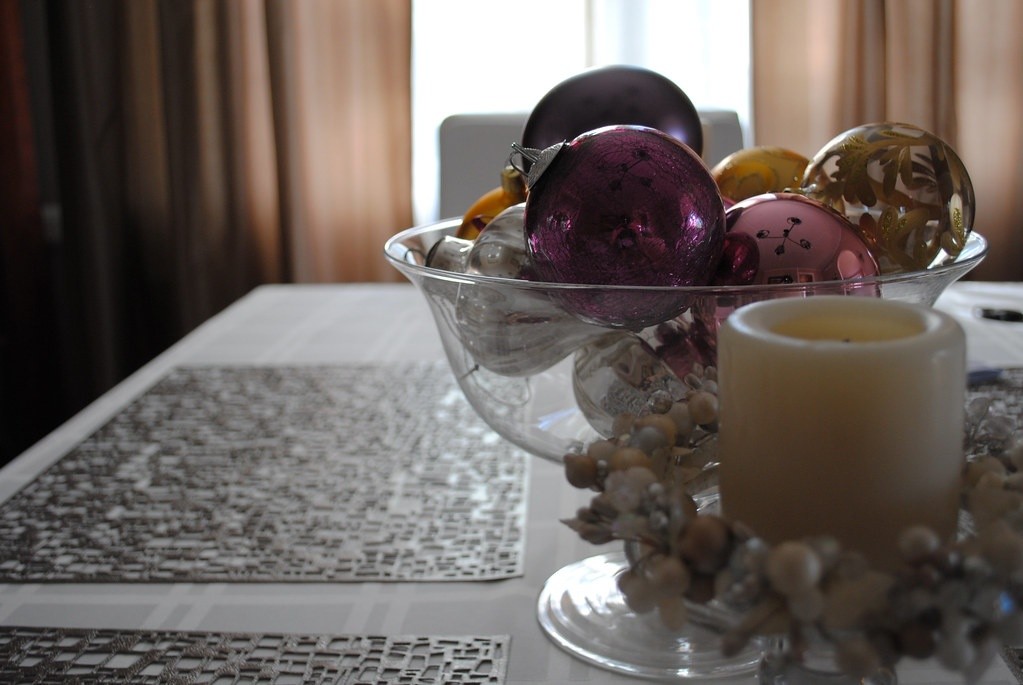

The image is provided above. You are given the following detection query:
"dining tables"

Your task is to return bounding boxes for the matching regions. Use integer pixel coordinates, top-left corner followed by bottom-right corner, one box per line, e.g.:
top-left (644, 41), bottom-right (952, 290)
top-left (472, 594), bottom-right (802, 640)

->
top-left (0, 286), bottom-right (1023, 685)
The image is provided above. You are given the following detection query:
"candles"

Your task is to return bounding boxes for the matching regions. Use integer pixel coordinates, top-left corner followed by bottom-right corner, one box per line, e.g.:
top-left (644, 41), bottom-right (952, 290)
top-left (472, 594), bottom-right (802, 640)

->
top-left (714, 293), bottom-right (968, 561)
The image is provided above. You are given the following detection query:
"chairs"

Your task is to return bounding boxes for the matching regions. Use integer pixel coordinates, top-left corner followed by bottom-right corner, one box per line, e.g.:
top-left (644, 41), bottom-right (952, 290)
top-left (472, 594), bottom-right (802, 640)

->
top-left (436, 111), bottom-right (744, 226)
